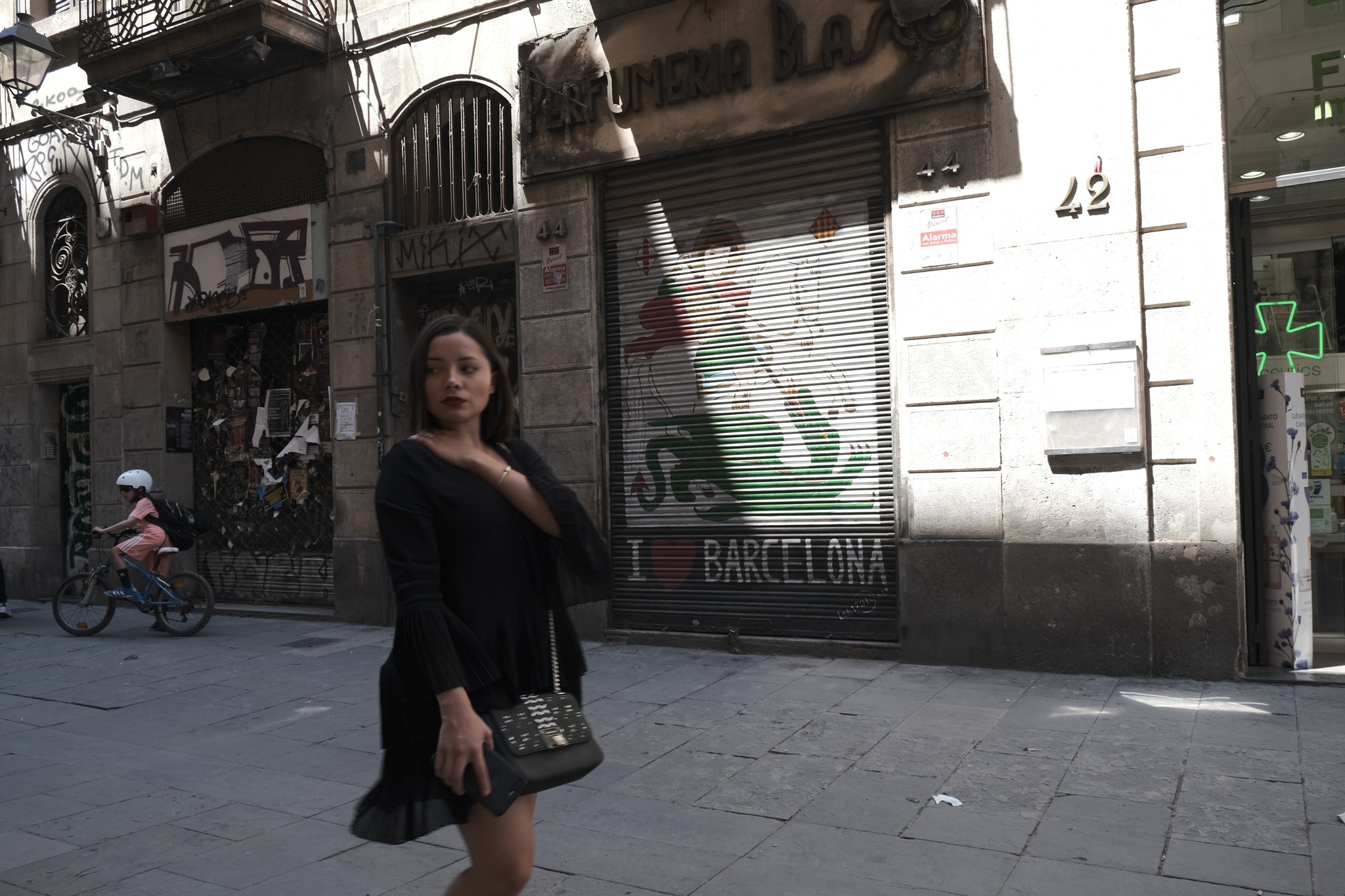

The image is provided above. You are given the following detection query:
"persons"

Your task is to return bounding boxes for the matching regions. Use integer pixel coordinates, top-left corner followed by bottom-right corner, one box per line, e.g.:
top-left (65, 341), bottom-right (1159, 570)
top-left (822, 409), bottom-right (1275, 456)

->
top-left (91, 469), bottom-right (172, 631)
top-left (349, 314), bottom-right (612, 896)
top-left (0, 562), bottom-right (12, 618)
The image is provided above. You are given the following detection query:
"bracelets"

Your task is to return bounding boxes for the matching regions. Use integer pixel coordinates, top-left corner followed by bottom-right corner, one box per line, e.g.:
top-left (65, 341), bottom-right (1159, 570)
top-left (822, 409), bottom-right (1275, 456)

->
top-left (495, 465), bottom-right (511, 489)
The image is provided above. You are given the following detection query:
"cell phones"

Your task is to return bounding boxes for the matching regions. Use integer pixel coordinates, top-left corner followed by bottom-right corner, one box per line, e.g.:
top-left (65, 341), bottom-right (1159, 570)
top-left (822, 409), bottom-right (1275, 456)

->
top-left (430, 743), bottom-right (528, 817)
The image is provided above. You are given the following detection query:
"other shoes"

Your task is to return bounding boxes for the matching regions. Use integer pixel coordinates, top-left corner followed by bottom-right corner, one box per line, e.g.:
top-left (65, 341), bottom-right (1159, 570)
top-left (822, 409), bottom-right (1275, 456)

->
top-left (0, 604), bottom-right (13, 617)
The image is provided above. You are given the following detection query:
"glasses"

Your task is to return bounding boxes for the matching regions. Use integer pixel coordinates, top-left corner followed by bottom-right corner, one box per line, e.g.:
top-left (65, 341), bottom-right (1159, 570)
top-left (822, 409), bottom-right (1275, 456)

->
top-left (119, 485), bottom-right (133, 493)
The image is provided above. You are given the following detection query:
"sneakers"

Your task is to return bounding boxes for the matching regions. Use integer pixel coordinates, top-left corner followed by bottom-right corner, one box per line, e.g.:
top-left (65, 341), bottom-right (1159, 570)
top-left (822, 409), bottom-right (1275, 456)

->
top-left (104, 586), bottom-right (133, 598)
top-left (147, 618), bottom-right (169, 630)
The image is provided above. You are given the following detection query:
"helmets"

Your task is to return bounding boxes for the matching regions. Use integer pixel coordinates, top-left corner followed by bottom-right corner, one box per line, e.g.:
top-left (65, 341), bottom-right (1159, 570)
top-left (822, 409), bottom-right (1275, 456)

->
top-left (116, 469), bottom-right (153, 493)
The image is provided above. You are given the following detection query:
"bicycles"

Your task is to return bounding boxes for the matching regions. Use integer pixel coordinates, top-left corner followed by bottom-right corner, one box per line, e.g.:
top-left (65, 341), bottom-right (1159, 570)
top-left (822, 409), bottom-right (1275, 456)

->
top-left (52, 526), bottom-right (215, 636)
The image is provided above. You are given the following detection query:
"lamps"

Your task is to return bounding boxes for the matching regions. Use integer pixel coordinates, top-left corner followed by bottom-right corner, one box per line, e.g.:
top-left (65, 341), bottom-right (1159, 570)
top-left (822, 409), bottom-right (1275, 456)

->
top-left (0, 13), bottom-right (105, 158)
top-left (239, 31), bottom-right (271, 67)
top-left (150, 58), bottom-right (181, 82)
top-left (83, 82), bottom-right (118, 108)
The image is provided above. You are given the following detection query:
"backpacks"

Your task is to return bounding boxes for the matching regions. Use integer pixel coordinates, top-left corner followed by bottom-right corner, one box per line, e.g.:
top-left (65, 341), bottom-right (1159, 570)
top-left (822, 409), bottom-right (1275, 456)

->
top-left (138, 494), bottom-right (196, 551)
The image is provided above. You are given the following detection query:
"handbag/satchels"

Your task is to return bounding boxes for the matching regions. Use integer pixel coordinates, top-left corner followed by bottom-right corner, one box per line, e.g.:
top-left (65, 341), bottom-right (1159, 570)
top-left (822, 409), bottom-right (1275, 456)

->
top-left (483, 691), bottom-right (603, 796)
